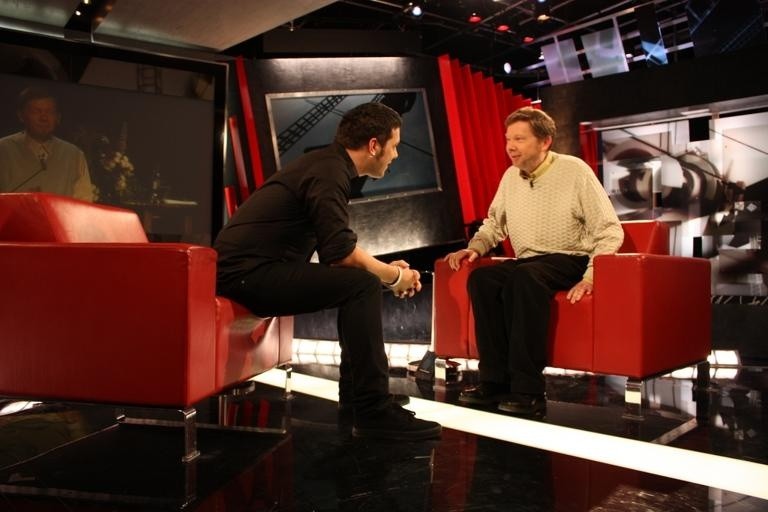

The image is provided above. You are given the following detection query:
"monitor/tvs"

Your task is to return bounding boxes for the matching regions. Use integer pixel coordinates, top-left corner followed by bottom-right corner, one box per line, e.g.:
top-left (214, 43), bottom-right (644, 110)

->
top-left (0, 23), bottom-right (230, 247)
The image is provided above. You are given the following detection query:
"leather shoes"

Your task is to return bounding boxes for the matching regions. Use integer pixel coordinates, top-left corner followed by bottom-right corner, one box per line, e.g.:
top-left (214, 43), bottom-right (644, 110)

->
top-left (338, 392), bottom-right (442, 440)
top-left (458, 384), bottom-right (548, 415)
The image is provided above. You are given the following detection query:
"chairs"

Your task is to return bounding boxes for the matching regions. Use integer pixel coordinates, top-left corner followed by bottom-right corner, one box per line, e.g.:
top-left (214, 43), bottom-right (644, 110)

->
top-left (433, 222), bottom-right (712, 424)
top-left (0, 192), bottom-right (295, 465)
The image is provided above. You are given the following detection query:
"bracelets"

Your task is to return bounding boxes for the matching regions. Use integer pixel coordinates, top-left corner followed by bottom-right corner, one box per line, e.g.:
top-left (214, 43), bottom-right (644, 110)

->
top-left (388, 265), bottom-right (404, 289)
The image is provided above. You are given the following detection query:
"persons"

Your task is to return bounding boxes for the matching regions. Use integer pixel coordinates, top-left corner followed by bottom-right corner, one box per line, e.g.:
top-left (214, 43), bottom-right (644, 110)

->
top-left (0, 86), bottom-right (93, 207)
top-left (214, 102), bottom-right (442, 441)
top-left (444, 106), bottom-right (624, 416)
